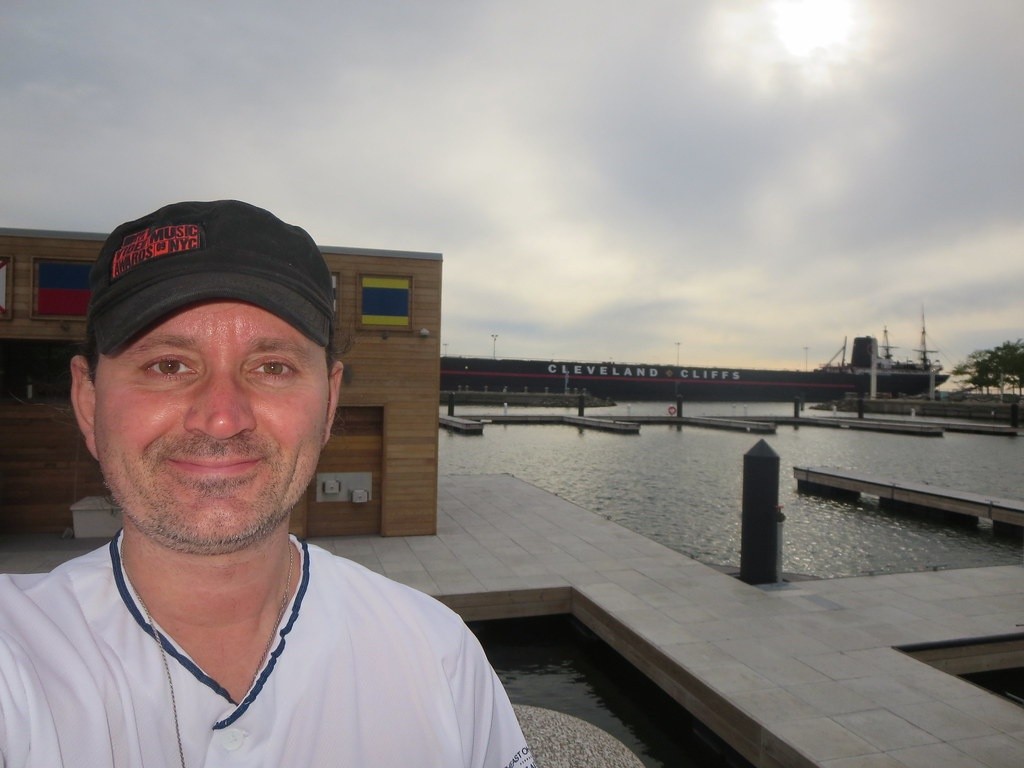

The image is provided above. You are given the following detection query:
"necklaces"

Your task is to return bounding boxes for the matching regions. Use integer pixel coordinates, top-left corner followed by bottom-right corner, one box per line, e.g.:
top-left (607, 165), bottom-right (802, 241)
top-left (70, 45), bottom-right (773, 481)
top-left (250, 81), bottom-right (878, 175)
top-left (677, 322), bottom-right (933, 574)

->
top-left (117, 536), bottom-right (292, 768)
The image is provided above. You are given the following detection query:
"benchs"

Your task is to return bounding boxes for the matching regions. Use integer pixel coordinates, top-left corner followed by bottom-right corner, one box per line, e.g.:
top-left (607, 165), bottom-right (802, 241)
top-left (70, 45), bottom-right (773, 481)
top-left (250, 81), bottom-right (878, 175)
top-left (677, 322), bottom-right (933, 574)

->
top-left (70, 496), bottom-right (121, 538)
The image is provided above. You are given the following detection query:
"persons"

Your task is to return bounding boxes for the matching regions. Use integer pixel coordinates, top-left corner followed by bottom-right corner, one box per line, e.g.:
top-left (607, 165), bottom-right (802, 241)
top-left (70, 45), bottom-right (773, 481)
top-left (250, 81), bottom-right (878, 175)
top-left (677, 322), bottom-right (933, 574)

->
top-left (2, 200), bottom-right (540, 768)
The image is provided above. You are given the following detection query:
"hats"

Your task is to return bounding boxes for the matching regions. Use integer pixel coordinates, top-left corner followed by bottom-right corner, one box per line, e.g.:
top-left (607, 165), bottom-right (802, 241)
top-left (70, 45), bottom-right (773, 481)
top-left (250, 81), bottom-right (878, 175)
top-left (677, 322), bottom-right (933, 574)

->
top-left (84, 200), bottom-right (334, 363)
